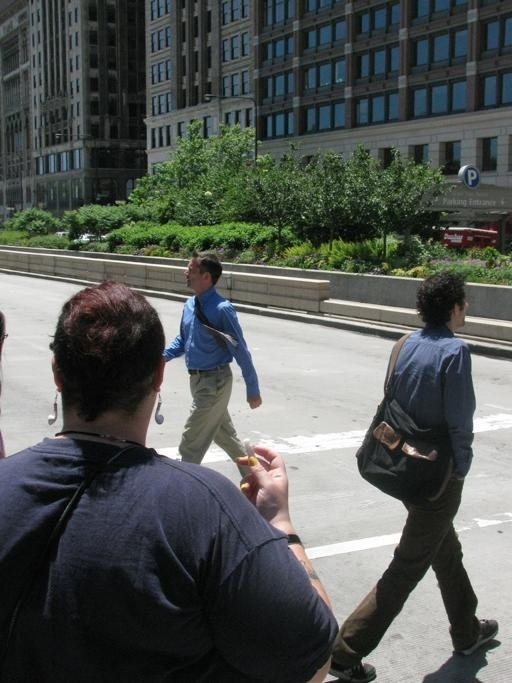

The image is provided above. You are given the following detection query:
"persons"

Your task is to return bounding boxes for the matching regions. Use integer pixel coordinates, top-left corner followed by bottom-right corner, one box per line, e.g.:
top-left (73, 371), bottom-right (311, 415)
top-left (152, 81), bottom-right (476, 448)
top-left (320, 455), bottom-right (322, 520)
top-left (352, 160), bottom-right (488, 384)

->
top-left (0, 310), bottom-right (9, 459)
top-left (0, 278), bottom-right (341, 681)
top-left (159, 251), bottom-right (262, 478)
top-left (326, 268), bottom-right (501, 683)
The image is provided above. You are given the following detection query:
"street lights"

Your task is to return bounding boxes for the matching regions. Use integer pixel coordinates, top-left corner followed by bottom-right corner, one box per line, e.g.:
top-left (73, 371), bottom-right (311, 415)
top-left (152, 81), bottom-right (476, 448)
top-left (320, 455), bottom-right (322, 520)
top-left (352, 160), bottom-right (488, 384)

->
top-left (56, 132), bottom-right (101, 201)
top-left (204, 92), bottom-right (258, 164)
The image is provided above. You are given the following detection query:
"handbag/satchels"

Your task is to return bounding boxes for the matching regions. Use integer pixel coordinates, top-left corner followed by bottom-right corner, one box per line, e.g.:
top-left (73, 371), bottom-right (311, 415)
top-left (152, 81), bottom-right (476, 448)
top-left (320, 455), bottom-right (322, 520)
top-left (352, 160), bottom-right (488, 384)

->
top-left (355, 393), bottom-right (450, 501)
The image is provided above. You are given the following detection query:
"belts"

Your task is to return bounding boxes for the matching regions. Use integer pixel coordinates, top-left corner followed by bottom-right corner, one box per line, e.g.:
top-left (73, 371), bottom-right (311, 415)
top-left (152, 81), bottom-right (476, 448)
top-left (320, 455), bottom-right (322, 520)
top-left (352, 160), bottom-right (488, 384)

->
top-left (187, 368), bottom-right (205, 374)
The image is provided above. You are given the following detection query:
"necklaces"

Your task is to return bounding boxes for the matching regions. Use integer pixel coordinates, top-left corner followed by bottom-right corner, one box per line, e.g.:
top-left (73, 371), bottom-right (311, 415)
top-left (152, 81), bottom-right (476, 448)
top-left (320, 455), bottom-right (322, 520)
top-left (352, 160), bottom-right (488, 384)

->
top-left (54, 430), bottom-right (148, 448)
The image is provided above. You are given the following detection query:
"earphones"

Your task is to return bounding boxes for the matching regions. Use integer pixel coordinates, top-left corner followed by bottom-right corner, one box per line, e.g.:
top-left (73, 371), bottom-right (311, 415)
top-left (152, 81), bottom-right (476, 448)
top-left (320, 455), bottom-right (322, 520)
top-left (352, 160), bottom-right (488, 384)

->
top-left (47, 403), bottom-right (59, 425)
top-left (155, 400), bottom-right (164, 427)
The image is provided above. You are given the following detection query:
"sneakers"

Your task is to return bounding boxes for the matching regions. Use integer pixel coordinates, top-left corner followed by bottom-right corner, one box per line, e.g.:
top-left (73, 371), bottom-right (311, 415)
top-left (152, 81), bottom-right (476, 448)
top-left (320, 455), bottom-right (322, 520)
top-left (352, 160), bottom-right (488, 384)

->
top-left (455, 620), bottom-right (498, 656)
top-left (329, 662), bottom-right (376, 682)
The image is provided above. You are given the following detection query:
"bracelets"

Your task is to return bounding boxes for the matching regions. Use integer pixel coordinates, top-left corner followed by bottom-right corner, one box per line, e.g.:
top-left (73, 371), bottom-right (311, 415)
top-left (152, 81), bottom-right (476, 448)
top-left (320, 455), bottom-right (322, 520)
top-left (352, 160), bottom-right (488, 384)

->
top-left (283, 533), bottom-right (303, 544)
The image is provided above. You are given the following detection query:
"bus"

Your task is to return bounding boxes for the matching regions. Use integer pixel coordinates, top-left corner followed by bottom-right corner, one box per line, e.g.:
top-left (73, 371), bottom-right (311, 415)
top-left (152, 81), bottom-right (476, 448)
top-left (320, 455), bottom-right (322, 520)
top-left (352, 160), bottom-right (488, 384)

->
top-left (429, 227), bottom-right (500, 252)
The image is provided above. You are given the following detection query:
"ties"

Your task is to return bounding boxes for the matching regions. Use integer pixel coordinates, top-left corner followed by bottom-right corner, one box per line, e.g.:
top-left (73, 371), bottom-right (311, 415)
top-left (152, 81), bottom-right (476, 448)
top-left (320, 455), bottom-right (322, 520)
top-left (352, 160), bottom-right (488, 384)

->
top-left (194, 296), bottom-right (238, 351)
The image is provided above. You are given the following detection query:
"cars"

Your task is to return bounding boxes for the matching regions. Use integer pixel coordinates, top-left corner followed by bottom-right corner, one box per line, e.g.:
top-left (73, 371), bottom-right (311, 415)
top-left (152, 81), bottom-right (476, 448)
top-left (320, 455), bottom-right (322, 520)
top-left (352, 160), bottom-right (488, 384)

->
top-left (55, 231), bottom-right (107, 245)
